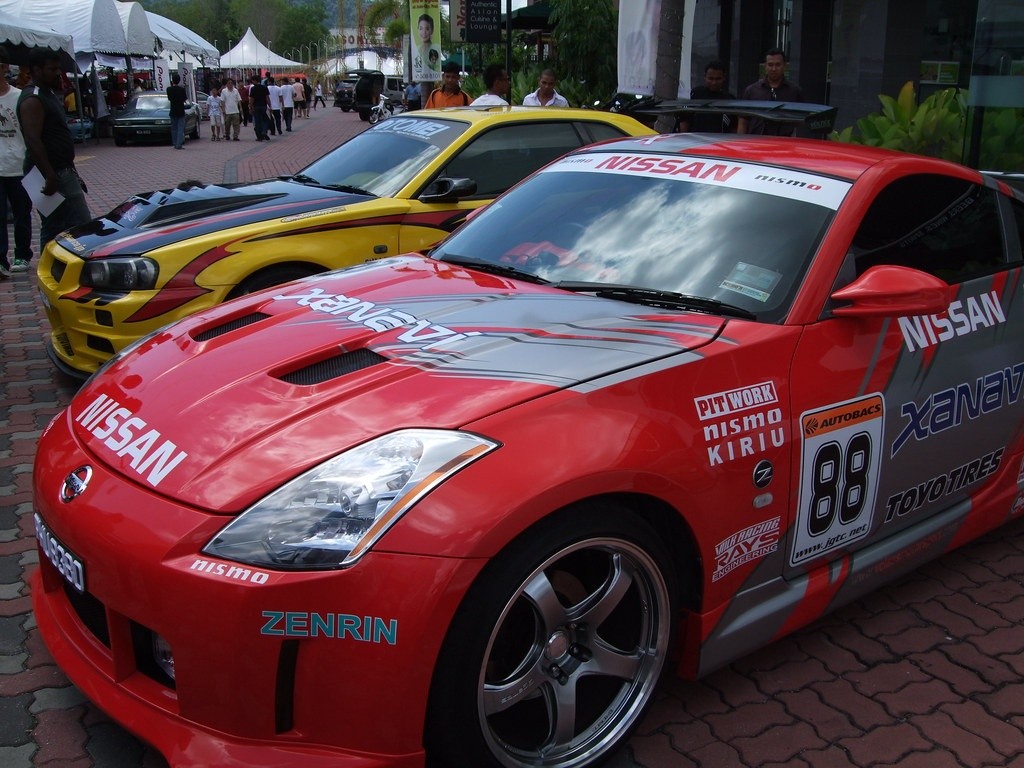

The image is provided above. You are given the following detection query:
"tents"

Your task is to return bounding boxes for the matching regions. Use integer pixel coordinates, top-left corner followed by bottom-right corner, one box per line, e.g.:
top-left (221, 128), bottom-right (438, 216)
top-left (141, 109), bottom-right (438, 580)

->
top-left (0, 0), bottom-right (221, 146)
top-left (195, 27), bottom-right (308, 69)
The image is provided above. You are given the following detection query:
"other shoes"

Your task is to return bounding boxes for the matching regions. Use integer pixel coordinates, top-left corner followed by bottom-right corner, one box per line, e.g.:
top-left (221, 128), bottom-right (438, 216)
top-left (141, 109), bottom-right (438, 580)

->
top-left (226, 136), bottom-right (230, 140)
top-left (271, 132), bottom-right (275, 135)
top-left (286, 128), bottom-right (291, 131)
top-left (256, 138), bottom-right (261, 141)
top-left (278, 129), bottom-right (282, 134)
top-left (211, 135), bottom-right (216, 141)
top-left (233, 138), bottom-right (239, 141)
top-left (216, 137), bottom-right (220, 141)
top-left (263, 133), bottom-right (270, 140)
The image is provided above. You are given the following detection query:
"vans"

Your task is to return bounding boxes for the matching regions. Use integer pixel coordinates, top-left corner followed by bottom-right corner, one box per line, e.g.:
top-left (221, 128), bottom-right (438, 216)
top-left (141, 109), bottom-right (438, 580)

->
top-left (345, 69), bottom-right (407, 120)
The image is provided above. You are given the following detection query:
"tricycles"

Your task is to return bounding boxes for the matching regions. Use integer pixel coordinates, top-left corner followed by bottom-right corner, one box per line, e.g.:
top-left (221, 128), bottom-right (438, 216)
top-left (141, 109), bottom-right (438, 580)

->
top-left (583, 92), bottom-right (839, 142)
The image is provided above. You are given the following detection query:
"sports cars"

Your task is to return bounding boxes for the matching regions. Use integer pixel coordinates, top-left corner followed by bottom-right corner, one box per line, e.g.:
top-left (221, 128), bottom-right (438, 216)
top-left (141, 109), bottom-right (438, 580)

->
top-left (37, 101), bottom-right (664, 376)
top-left (28, 131), bottom-right (1024, 768)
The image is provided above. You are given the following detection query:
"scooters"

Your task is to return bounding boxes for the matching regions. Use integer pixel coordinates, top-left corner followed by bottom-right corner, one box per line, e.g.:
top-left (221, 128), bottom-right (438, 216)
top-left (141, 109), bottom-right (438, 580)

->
top-left (368, 92), bottom-right (395, 124)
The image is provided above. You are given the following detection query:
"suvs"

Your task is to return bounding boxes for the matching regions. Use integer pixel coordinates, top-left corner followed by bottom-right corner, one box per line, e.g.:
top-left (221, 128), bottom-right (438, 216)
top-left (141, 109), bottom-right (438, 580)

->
top-left (332, 77), bottom-right (357, 113)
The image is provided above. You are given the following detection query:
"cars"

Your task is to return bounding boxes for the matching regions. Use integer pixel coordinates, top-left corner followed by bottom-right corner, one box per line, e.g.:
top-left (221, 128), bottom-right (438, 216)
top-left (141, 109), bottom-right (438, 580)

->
top-left (112, 90), bottom-right (224, 146)
top-left (65, 114), bottom-right (95, 143)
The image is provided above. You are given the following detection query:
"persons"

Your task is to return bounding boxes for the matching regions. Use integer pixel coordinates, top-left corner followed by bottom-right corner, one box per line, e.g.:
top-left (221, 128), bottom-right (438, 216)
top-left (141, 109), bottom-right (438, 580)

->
top-left (167, 74), bottom-right (188, 149)
top-left (737, 48), bottom-right (803, 136)
top-left (424, 61), bottom-right (474, 109)
top-left (0, 46), bottom-right (91, 278)
top-left (680, 61), bottom-right (738, 133)
top-left (523, 69), bottom-right (569, 108)
top-left (470, 64), bottom-right (510, 106)
top-left (206, 72), bottom-right (326, 141)
top-left (417, 14), bottom-right (441, 72)
top-left (405, 79), bottom-right (421, 111)
top-left (131, 78), bottom-right (142, 93)
top-left (426, 49), bottom-right (440, 72)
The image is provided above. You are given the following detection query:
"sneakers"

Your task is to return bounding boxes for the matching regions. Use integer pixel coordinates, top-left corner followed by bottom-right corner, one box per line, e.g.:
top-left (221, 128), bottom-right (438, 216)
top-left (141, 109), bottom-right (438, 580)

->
top-left (10, 259), bottom-right (31, 270)
top-left (0, 265), bottom-right (10, 276)
